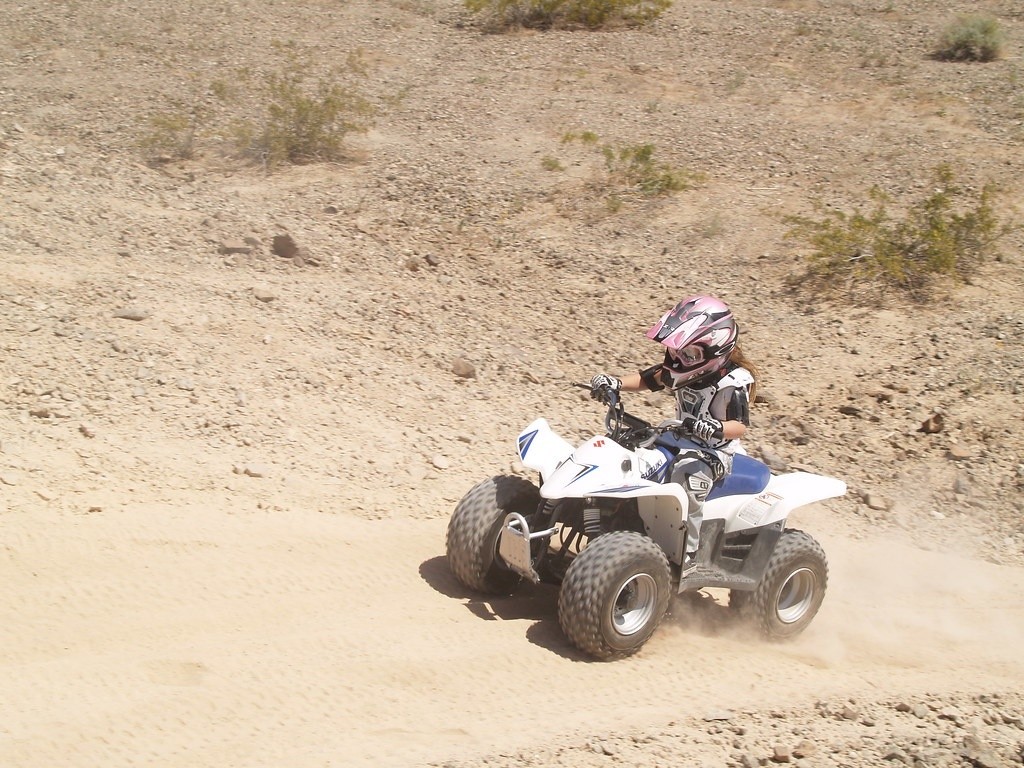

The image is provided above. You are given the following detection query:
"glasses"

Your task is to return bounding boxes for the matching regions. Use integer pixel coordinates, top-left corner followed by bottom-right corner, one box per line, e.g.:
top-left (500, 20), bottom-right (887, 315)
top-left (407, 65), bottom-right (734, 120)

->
top-left (667, 345), bottom-right (705, 367)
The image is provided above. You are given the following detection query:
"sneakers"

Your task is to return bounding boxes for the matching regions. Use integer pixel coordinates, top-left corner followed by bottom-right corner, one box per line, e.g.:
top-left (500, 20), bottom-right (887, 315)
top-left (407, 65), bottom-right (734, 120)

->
top-left (682, 553), bottom-right (697, 578)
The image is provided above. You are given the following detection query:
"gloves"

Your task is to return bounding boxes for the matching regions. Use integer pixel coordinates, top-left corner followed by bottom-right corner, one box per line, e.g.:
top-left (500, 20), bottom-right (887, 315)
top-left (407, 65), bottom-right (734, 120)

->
top-left (589, 373), bottom-right (623, 402)
top-left (687, 418), bottom-right (723, 446)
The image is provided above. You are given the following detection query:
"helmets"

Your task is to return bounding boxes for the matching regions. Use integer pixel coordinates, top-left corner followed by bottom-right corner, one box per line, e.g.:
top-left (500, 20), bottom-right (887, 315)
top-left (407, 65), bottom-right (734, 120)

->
top-left (646, 296), bottom-right (738, 391)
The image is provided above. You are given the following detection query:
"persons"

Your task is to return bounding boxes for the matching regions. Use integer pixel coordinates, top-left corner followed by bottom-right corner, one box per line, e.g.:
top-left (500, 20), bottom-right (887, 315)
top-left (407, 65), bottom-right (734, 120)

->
top-left (590, 294), bottom-right (761, 578)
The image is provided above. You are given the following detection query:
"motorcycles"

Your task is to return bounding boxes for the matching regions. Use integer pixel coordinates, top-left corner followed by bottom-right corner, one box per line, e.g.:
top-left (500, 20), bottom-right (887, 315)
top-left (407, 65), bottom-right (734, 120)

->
top-left (445, 382), bottom-right (848, 664)
